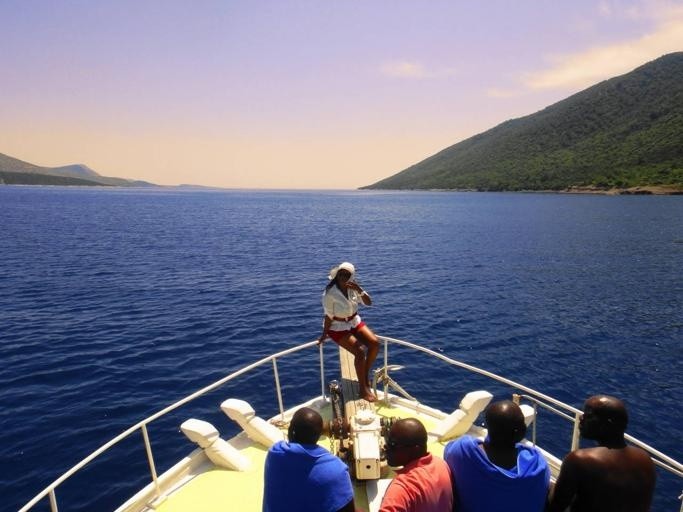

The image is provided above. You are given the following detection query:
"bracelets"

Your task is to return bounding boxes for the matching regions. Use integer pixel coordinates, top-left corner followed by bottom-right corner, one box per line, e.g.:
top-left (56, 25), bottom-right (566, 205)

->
top-left (358, 290), bottom-right (366, 297)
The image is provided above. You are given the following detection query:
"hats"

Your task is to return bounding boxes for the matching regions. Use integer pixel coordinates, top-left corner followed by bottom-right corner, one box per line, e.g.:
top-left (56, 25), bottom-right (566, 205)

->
top-left (330, 261), bottom-right (356, 282)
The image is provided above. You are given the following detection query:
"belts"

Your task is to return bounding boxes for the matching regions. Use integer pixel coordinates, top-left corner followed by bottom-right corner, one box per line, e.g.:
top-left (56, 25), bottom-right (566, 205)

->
top-left (333, 311), bottom-right (357, 322)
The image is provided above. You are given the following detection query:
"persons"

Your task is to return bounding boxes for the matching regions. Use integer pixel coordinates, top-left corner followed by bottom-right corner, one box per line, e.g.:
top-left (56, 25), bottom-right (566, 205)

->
top-left (317, 261), bottom-right (380, 402)
top-left (379, 418), bottom-right (454, 512)
top-left (444, 400), bottom-right (550, 512)
top-left (549, 395), bottom-right (657, 512)
top-left (262, 407), bottom-right (356, 512)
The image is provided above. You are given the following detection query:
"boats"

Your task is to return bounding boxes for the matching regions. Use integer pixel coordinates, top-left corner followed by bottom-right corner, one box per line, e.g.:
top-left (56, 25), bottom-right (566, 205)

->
top-left (16, 331), bottom-right (682, 510)
